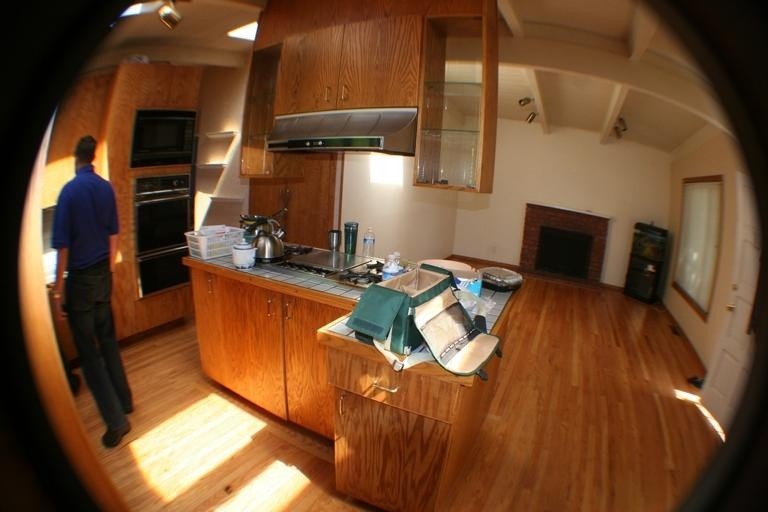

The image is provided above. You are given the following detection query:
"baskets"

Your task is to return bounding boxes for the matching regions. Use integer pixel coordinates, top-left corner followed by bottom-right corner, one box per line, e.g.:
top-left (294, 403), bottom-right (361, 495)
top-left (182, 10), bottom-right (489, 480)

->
top-left (184, 226), bottom-right (247, 260)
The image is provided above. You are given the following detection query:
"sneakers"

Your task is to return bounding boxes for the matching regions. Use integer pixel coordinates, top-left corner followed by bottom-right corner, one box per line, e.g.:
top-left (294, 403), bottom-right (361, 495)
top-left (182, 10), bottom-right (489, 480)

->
top-left (102, 405), bottom-right (133, 447)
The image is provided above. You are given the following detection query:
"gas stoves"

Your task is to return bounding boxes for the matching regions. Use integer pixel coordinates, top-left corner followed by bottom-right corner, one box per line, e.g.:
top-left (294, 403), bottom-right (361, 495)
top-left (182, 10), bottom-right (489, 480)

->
top-left (264, 244), bottom-right (418, 289)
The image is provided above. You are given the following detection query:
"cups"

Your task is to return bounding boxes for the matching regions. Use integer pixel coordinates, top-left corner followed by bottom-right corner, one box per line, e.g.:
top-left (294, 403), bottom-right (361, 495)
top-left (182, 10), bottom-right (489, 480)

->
top-left (344, 222), bottom-right (358, 254)
top-left (328, 229), bottom-right (341, 252)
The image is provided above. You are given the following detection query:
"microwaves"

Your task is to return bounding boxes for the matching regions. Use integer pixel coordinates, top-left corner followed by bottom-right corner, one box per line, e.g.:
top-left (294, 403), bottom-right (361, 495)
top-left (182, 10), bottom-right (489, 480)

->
top-left (132, 110), bottom-right (197, 166)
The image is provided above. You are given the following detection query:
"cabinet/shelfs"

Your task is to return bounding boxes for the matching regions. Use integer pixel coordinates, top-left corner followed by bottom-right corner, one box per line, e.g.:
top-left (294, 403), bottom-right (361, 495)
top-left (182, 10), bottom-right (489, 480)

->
top-left (230, 281), bottom-right (348, 442)
top-left (413, 6), bottom-right (498, 193)
top-left (238, 43), bottom-right (306, 180)
top-left (188, 268), bottom-right (227, 386)
top-left (276, 16), bottom-right (419, 118)
top-left (315, 274), bottom-right (530, 512)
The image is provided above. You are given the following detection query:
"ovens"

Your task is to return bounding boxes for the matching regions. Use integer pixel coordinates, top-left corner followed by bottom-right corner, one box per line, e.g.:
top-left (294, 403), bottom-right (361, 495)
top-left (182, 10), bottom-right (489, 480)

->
top-left (132, 173), bottom-right (193, 298)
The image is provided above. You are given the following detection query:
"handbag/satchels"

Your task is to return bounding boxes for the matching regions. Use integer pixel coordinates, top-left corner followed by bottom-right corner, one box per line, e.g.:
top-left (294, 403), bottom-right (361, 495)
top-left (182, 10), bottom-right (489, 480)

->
top-left (346, 264), bottom-right (500, 375)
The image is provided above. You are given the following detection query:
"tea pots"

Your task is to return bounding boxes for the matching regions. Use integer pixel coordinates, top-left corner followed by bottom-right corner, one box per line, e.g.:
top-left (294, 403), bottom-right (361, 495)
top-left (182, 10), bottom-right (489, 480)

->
top-left (251, 228), bottom-right (287, 264)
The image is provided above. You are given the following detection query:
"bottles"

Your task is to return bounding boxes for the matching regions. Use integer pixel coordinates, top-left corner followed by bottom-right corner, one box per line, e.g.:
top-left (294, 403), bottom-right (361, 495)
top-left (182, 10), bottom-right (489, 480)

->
top-left (362, 227), bottom-right (376, 258)
top-left (230, 237), bottom-right (258, 269)
top-left (382, 252), bottom-right (401, 275)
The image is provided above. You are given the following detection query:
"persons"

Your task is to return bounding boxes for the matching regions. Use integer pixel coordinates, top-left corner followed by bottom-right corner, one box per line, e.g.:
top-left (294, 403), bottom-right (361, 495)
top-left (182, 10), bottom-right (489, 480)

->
top-left (51, 136), bottom-right (134, 448)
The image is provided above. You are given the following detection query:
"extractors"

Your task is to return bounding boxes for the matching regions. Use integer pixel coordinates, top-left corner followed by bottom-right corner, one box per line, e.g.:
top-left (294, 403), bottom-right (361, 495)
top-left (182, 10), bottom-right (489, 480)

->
top-left (264, 103), bottom-right (419, 159)
